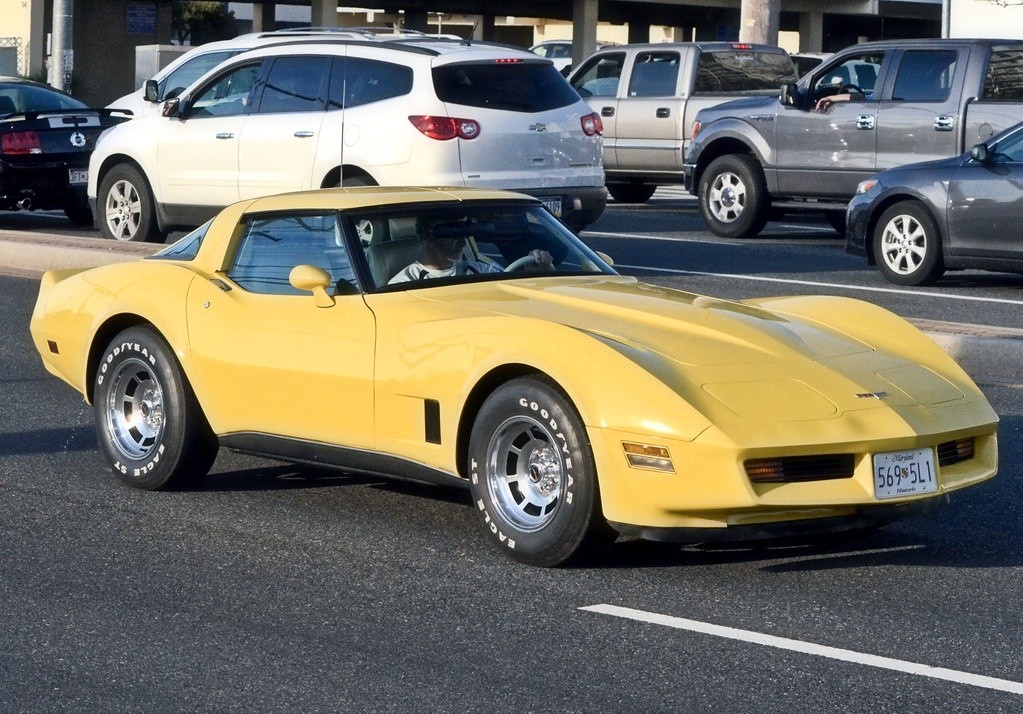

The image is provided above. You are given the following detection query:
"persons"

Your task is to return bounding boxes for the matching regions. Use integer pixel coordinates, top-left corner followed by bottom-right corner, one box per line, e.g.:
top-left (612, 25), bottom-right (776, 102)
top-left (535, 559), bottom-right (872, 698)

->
top-left (814, 94), bottom-right (872, 113)
top-left (386, 216), bottom-right (553, 286)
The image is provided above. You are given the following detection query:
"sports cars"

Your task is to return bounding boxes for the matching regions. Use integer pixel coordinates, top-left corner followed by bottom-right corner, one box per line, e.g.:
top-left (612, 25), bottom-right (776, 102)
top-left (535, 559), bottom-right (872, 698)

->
top-left (30, 42), bottom-right (1000, 568)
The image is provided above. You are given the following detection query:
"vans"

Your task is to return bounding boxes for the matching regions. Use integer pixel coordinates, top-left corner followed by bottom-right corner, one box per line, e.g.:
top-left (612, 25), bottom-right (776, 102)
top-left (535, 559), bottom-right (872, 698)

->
top-left (87, 27), bottom-right (607, 269)
top-left (567, 42), bottom-right (802, 203)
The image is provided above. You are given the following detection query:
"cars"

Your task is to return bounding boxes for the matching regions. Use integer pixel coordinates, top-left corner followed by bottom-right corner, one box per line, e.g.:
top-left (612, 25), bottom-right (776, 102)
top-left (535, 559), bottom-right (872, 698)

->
top-left (843, 121), bottom-right (1023, 286)
top-left (0, 76), bottom-right (135, 230)
top-left (790, 52), bottom-right (835, 78)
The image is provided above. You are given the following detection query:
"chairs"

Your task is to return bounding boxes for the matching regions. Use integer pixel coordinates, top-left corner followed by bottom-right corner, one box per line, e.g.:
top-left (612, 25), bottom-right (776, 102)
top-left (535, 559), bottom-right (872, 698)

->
top-left (368, 239), bottom-right (419, 288)
top-left (0, 95), bottom-right (17, 115)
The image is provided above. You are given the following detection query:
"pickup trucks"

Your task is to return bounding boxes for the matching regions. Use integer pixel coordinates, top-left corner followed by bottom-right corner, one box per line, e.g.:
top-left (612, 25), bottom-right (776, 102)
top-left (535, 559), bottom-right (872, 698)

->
top-left (684, 38), bottom-right (1023, 238)
